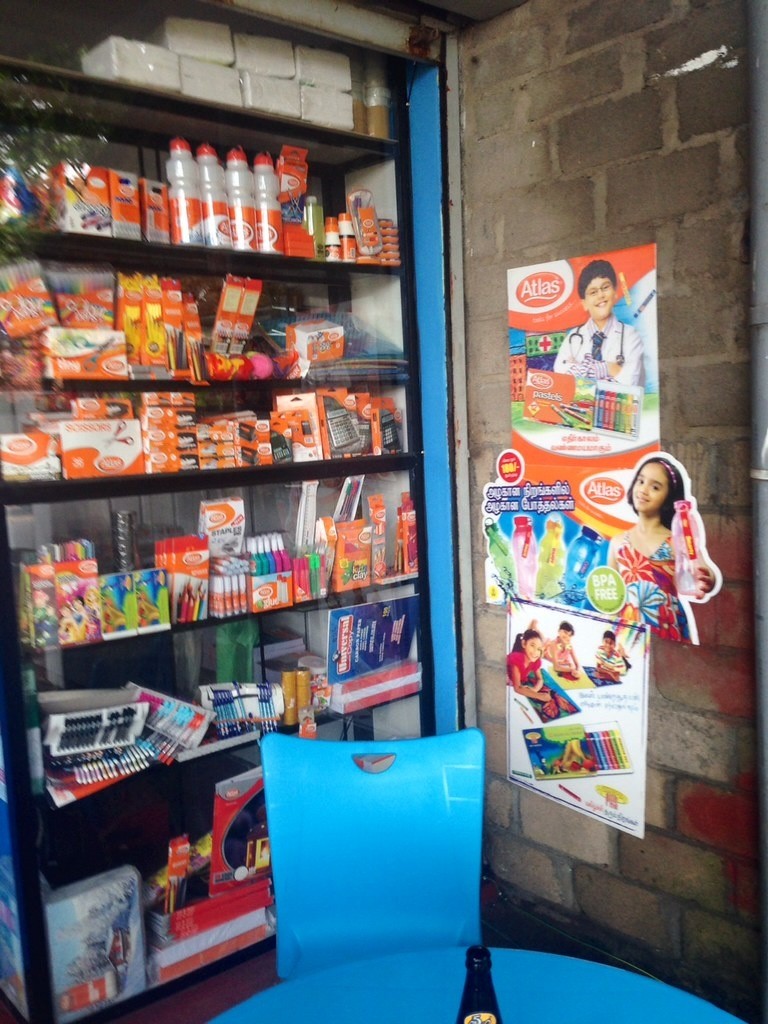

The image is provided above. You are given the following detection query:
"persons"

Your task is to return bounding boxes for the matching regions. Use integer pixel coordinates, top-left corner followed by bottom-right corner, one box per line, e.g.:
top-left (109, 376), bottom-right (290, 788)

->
top-left (506, 629), bottom-right (552, 702)
top-left (528, 619), bottom-right (582, 679)
top-left (607, 456), bottom-right (716, 646)
top-left (595, 630), bottom-right (627, 682)
top-left (553, 260), bottom-right (645, 387)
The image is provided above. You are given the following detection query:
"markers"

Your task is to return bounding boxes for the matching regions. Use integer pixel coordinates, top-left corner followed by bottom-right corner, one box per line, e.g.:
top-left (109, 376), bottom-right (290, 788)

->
top-left (557, 783), bottom-right (582, 803)
top-left (514, 697), bottom-right (534, 724)
top-left (293, 553), bottom-right (326, 603)
top-left (584, 729), bottom-right (631, 770)
top-left (207, 556), bottom-right (249, 618)
top-left (512, 770), bottom-right (532, 779)
top-left (618, 271), bottom-right (656, 318)
top-left (550, 389), bottom-right (641, 438)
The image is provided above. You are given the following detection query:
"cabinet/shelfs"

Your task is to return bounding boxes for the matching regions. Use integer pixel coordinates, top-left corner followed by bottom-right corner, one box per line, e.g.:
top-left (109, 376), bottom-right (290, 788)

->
top-left (0, 43), bottom-right (439, 1024)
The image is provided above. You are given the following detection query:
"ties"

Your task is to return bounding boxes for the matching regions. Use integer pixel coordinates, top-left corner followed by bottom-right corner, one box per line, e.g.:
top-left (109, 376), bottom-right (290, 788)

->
top-left (591, 332), bottom-right (605, 362)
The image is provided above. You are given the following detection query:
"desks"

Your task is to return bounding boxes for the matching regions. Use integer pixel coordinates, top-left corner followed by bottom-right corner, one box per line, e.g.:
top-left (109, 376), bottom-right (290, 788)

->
top-left (201, 945), bottom-right (749, 1023)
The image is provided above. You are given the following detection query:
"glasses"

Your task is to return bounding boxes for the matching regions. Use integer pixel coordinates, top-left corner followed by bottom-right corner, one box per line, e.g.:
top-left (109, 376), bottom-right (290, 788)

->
top-left (584, 282), bottom-right (613, 296)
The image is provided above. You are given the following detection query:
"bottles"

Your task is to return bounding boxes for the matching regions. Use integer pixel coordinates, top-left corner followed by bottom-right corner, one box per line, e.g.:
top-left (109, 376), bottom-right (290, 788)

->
top-left (484, 517), bottom-right (515, 582)
top-left (253, 150), bottom-right (283, 254)
top-left (455, 945), bottom-right (504, 1024)
top-left (346, 185), bottom-right (382, 255)
top-left (337, 212), bottom-right (357, 263)
top-left (195, 140), bottom-right (231, 249)
top-left (303, 194), bottom-right (327, 262)
top-left (563, 525), bottom-right (601, 601)
top-left (671, 499), bottom-right (708, 596)
top-left (536, 513), bottom-right (566, 598)
top-left (225, 144), bottom-right (256, 253)
top-left (166, 135), bottom-right (206, 247)
top-left (323, 217), bottom-right (342, 262)
top-left (511, 516), bottom-right (538, 597)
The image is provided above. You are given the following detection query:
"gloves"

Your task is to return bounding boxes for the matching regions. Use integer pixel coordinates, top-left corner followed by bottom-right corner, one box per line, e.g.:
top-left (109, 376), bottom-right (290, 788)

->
top-left (568, 354), bottom-right (610, 380)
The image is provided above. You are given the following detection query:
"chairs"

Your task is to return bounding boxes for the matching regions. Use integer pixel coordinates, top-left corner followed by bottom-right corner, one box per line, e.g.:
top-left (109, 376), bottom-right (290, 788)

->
top-left (258, 724), bottom-right (485, 982)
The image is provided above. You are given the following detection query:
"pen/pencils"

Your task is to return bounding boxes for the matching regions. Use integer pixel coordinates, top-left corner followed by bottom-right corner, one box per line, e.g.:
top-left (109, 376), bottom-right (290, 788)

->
top-left (336, 479), bottom-right (359, 522)
top-left (123, 302), bottom-right (212, 382)
top-left (177, 577), bottom-right (207, 622)
top-left (394, 492), bottom-right (418, 575)
top-left (44, 691), bottom-right (206, 785)
top-left (0, 257), bottom-right (115, 294)
top-left (211, 681), bottom-right (279, 748)
top-left (18, 538), bottom-right (94, 566)
top-left (214, 330), bottom-right (250, 355)
top-left (165, 872), bottom-right (188, 914)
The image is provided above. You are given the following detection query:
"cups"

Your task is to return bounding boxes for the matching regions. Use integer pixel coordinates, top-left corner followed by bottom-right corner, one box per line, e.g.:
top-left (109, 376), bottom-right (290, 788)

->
top-left (349, 82), bottom-right (367, 133)
top-left (366, 87), bottom-right (391, 140)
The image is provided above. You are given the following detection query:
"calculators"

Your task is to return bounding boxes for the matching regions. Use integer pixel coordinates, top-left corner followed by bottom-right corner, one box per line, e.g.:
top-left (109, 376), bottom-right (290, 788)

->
top-left (382, 413), bottom-right (399, 447)
top-left (301, 420), bottom-right (312, 435)
top-left (269, 432), bottom-right (291, 461)
top-left (327, 407), bottom-right (372, 454)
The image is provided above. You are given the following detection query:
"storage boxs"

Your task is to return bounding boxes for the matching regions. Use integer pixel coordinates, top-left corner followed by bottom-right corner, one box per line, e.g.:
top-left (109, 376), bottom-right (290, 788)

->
top-left (1, 145), bottom-right (405, 655)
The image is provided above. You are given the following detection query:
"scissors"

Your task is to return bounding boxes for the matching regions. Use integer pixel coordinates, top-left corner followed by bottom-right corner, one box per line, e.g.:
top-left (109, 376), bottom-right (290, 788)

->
top-left (99, 421), bottom-right (135, 451)
top-left (73, 336), bottom-right (125, 371)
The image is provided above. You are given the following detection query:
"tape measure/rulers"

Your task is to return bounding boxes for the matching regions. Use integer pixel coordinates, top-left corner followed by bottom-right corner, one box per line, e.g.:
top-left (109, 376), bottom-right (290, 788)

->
top-left (300, 483), bottom-right (318, 556)
